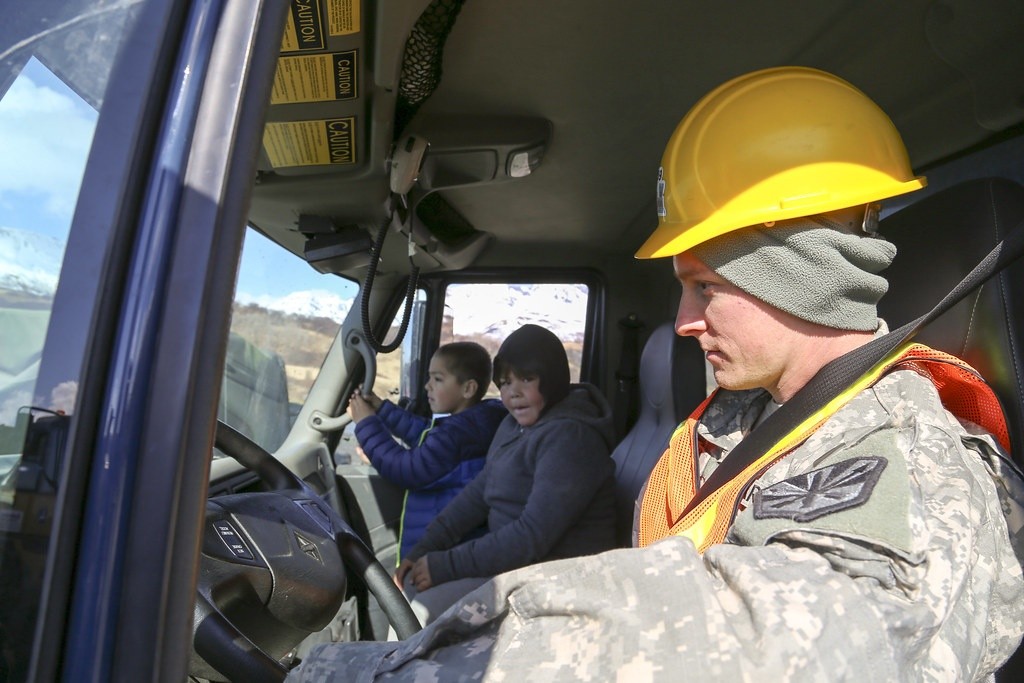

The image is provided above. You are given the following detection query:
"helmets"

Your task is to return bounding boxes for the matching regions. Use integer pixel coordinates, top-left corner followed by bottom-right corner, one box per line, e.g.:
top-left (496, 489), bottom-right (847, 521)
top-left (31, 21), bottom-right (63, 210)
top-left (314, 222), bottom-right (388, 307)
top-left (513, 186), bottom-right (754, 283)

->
top-left (633, 64), bottom-right (927, 259)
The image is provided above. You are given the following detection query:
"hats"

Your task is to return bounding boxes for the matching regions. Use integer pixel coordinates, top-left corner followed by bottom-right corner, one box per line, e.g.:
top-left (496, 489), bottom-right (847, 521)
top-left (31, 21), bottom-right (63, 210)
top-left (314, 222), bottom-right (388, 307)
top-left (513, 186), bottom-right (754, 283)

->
top-left (498, 325), bottom-right (570, 394)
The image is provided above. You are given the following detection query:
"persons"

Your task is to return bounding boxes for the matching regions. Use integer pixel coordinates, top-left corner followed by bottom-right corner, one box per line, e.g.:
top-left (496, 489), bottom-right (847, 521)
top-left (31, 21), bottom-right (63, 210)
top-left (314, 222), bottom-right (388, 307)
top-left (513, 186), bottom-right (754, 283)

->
top-left (383, 322), bottom-right (623, 648)
top-left (351, 339), bottom-right (510, 644)
top-left (283, 65), bottom-right (1024, 683)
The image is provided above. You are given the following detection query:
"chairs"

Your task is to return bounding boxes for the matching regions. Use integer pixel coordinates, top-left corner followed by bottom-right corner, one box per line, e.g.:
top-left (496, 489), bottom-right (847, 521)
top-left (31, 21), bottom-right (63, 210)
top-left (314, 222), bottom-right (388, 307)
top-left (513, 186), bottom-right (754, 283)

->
top-left (607, 324), bottom-right (706, 512)
top-left (876, 163), bottom-right (1024, 470)
